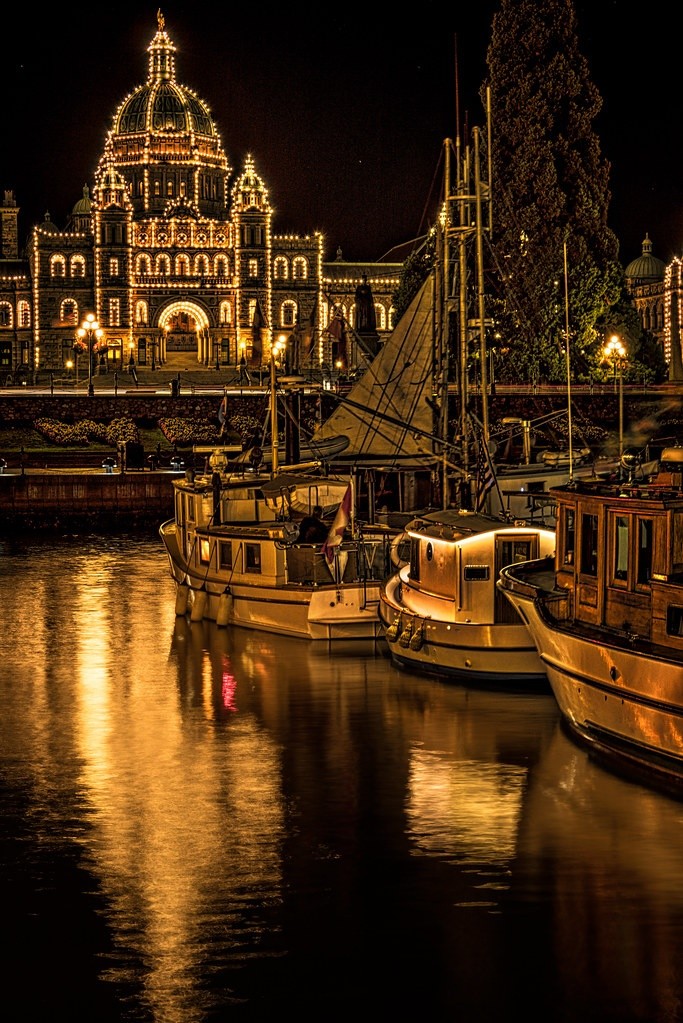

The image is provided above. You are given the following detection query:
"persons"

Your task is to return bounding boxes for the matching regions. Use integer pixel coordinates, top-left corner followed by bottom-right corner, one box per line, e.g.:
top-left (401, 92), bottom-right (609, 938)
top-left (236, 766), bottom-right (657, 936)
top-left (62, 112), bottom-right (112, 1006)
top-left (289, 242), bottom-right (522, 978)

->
top-left (291, 506), bottom-right (326, 548)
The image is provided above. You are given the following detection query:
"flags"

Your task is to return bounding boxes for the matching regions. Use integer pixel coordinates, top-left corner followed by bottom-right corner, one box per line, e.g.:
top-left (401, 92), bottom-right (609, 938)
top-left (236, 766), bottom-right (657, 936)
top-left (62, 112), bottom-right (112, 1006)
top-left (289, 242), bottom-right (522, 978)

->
top-left (321, 484), bottom-right (352, 563)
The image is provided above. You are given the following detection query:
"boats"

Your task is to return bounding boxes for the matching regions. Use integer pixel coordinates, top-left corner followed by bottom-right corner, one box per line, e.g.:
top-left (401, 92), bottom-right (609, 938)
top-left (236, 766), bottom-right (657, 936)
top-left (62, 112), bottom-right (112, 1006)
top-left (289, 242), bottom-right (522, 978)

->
top-left (158, 333), bottom-right (392, 641)
top-left (376, 501), bottom-right (551, 689)
top-left (234, 434), bottom-right (350, 465)
top-left (496, 440), bottom-right (683, 782)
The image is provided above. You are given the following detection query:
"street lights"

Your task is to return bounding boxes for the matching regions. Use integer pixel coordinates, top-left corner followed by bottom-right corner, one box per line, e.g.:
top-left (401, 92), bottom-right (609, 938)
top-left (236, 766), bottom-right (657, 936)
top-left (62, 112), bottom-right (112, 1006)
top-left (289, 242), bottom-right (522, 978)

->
top-left (272, 335), bottom-right (288, 387)
top-left (337, 363), bottom-right (342, 376)
top-left (77, 313), bottom-right (104, 396)
top-left (129, 343), bottom-right (135, 365)
top-left (601, 335), bottom-right (626, 392)
top-left (240, 342), bottom-right (246, 365)
top-left (67, 362), bottom-right (73, 374)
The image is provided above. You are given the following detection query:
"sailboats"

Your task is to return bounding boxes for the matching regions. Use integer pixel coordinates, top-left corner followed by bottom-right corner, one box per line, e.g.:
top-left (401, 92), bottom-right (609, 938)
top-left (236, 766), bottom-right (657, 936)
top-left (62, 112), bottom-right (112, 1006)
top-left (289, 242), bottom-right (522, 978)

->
top-left (306, 81), bottom-right (621, 523)
